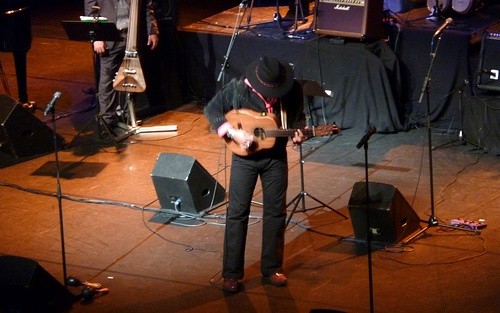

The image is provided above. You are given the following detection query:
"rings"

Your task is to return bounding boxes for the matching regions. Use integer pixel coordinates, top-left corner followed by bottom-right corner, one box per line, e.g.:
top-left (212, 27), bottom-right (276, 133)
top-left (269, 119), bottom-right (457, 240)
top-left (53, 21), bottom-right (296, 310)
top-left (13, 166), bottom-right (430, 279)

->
top-left (246, 144), bottom-right (250, 147)
top-left (246, 141), bottom-right (250, 143)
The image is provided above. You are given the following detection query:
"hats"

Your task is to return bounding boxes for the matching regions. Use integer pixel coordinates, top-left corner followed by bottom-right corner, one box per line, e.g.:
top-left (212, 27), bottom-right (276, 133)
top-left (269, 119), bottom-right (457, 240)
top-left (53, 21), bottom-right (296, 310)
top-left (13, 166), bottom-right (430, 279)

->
top-left (245, 54), bottom-right (294, 97)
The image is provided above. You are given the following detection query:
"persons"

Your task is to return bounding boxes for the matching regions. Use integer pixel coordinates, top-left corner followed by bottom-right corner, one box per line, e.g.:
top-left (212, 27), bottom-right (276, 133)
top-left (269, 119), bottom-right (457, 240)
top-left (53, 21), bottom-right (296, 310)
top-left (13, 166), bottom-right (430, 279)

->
top-left (202, 56), bottom-right (312, 292)
top-left (84, 0), bottom-right (159, 146)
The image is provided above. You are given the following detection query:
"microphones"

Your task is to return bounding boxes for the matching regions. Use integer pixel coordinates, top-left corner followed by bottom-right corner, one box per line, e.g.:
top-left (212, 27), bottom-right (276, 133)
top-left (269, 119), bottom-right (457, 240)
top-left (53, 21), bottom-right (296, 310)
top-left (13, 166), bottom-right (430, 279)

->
top-left (433, 17), bottom-right (453, 37)
top-left (44, 91), bottom-right (61, 116)
top-left (356, 125), bottom-right (377, 149)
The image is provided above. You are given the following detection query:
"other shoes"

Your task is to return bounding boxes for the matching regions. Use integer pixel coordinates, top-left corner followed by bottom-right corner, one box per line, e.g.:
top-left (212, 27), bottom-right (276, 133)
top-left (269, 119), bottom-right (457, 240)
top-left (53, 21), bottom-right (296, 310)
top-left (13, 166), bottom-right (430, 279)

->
top-left (224, 279), bottom-right (239, 291)
top-left (262, 273), bottom-right (287, 286)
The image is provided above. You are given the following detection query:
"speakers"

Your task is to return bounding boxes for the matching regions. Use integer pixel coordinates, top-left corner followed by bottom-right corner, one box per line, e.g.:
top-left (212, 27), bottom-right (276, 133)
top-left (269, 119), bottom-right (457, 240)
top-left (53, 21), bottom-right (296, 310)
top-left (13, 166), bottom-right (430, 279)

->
top-left (0, 91), bottom-right (66, 169)
top-left (151, 152), bottom-right (227, 216)
top-left (348, 181), bottom-right (422, 244)
top-left (0, 253), bottom-right (77, 313)
top-left (314, 0), bottom-right (384, 40)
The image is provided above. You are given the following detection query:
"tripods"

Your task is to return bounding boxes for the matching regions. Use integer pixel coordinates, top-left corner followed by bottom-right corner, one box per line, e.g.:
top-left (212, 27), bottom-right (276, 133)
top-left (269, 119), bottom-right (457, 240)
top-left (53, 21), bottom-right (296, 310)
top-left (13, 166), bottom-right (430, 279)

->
top-left (429, 71), bottom-right (487, 153)
top-left (283, 80), bottom-right (347, 233)
top-left (60, 21), bottom-right (122, 153)
top-left (401, 33), bottom-right (480, 245)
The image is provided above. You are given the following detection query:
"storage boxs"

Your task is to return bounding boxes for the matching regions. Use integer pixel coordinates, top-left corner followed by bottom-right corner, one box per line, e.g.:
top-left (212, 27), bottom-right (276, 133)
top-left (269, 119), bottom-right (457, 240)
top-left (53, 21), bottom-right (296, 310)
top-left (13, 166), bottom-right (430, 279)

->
top-left (313, 0), bottom-right (384, 41)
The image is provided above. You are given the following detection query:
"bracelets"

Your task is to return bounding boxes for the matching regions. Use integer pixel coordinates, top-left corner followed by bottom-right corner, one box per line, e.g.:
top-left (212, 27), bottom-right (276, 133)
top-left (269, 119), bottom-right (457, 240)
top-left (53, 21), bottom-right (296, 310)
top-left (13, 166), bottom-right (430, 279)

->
top-left (227, 128), bottom-right (235, 139)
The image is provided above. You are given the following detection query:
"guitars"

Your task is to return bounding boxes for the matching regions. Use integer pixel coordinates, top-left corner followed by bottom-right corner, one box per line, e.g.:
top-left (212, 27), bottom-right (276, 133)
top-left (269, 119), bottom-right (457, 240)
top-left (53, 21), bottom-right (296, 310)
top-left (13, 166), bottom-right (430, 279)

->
top-left (222, 107), bottom-right (340, 156)
top-left (113, 0), bottom-right (145, 93)
